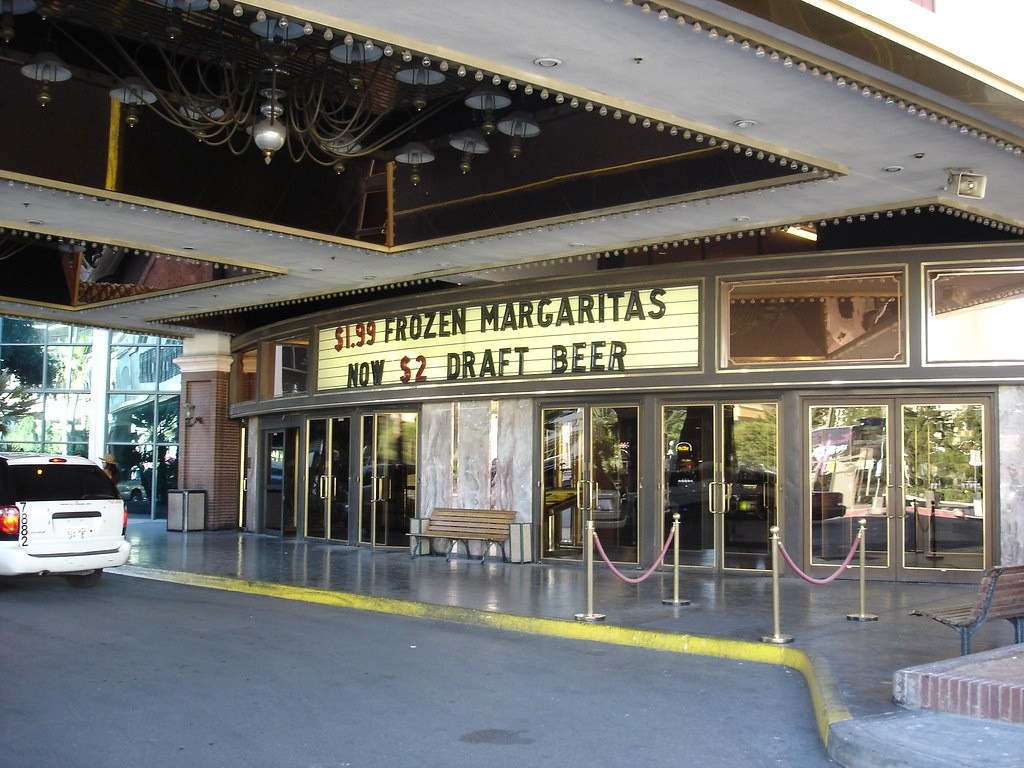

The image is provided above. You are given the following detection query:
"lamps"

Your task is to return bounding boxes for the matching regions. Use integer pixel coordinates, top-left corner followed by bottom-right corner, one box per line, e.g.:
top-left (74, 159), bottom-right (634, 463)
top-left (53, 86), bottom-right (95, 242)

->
top-left (0, 0), bottom-right (545, 187)
top-left (183, 401), bottom-right (202, 427)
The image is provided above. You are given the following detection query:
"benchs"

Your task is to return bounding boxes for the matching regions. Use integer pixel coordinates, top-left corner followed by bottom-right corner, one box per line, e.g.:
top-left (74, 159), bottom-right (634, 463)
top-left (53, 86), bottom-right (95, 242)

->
top-left (906, 564), bottom-right (1024, 657)
top-left (406, 507), bottom-right (518, 566)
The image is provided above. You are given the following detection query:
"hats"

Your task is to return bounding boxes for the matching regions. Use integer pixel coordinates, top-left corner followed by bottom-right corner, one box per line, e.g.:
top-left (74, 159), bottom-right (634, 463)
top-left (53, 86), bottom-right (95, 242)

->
top-left (99, 454), bottom-right (119, 464)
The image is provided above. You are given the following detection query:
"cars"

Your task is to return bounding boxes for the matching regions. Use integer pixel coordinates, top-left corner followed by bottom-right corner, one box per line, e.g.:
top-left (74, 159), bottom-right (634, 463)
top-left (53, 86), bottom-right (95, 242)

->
top-left (115, 481), bottom-right (150, 503)
top-left (725, 470), bottom-right (844, 524)
top-left (1, 452), bottom-right (132, 588)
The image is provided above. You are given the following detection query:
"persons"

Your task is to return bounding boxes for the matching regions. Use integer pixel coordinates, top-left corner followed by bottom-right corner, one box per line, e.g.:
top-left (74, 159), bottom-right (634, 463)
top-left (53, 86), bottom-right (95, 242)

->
top-left (97, 453), bottom-right (119, 487)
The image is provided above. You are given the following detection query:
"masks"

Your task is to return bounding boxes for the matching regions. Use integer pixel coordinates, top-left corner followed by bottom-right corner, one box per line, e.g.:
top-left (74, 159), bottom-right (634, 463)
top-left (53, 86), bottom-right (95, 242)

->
top-left (102, 463), bottom-right (107, 469)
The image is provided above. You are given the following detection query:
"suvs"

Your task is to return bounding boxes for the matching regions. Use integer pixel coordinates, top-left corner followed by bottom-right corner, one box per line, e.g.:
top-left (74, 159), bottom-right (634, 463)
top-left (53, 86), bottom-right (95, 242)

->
top-left (587, 464), bottom-right (628, 541)
